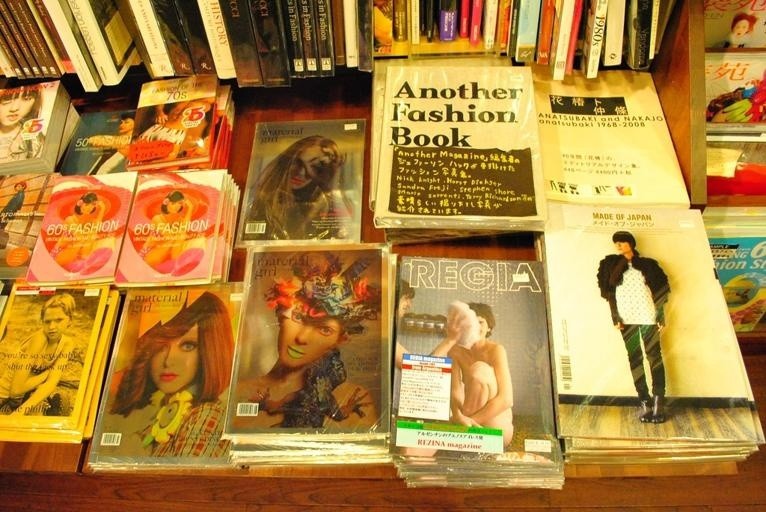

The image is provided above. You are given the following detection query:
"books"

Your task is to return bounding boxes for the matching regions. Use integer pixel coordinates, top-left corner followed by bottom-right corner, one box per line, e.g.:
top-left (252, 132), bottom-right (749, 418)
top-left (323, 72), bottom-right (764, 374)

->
top-left (702, 0), bottom-right (766, 332)
top-left (0, 60), bottom-right (766, 492)
top-left (0, 2), bottom-right (675, 92)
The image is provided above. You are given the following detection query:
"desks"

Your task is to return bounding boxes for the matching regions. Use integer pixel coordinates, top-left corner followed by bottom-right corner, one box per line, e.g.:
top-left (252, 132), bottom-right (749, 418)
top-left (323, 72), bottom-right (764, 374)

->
top-left (1, 0), bottom-right (765, 512)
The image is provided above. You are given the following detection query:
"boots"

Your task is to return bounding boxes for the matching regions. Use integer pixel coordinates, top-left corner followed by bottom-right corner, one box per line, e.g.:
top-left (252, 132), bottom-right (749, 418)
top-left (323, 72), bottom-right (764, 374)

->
top-left (652, 395), bottom-right (665, 423)
top-left (638, 396), bottom-right (653, 422)
